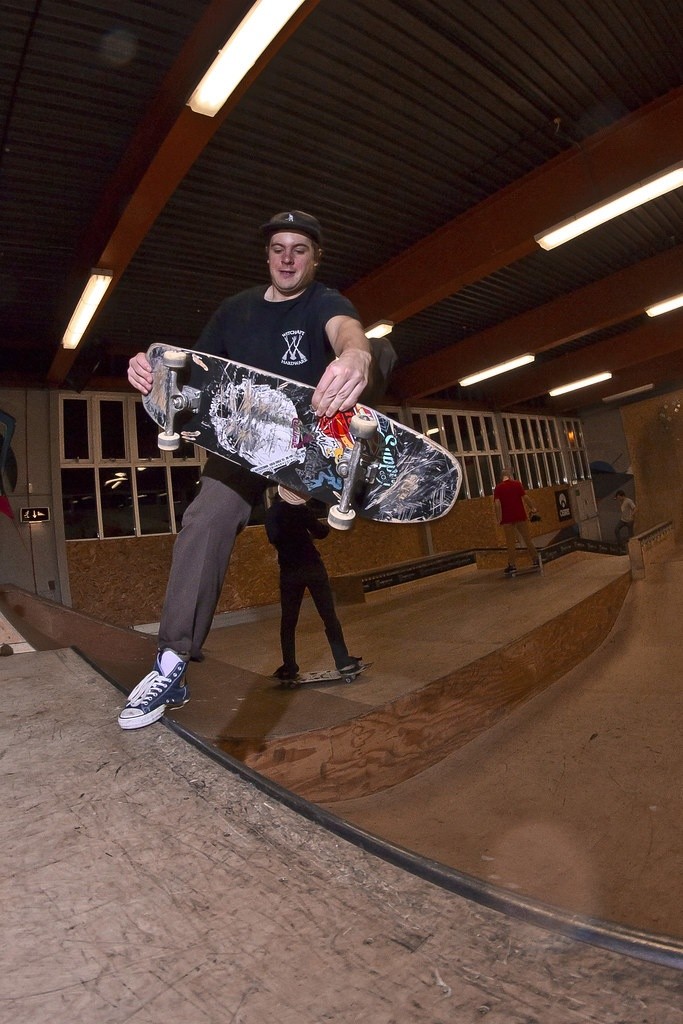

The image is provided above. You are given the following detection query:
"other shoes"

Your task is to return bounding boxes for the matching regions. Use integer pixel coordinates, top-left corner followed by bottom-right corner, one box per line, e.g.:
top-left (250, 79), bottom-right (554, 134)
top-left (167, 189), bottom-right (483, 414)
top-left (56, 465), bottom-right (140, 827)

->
top-left (532, 560), bottom-right (539, 567)
top-left (504, 565), bottom-right (515, 573)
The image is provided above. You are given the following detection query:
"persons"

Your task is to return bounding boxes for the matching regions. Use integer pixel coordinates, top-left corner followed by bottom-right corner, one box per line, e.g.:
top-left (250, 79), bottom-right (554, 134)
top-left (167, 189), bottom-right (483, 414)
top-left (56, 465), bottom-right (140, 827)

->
top-left (615, 490), bottom-right (637, 555)
top-left (494, 468), bottom-right (540, 574)
top-left (263, 491), bottom-right (363, 678)
top-left (116, 211), bottom-right (370, 728)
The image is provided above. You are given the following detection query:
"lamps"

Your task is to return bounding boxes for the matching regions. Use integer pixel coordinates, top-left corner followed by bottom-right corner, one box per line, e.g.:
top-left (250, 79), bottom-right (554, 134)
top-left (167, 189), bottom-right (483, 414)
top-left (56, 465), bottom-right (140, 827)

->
top-left (549, 370), bottom-right (613, 397)
top-left (458, 353), bottom-right (536, 387)
top-left (643, 290), bottom-right (683, 317)
top-left (365, 319), bottom-right (395, 339)
top-left (603, 384), bottom-right (654, 402)
top-left (59, 268), bottom-right (114, 349)
top-left (186, 1), bottom-right (305, 117)
top-left (534, 161), bottom-right (683, 251)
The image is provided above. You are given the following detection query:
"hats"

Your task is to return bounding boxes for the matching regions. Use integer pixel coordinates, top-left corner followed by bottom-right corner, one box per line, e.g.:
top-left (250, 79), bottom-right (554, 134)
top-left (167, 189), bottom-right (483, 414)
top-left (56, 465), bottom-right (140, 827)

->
top-left (257, 211), bottom-right (323, 245)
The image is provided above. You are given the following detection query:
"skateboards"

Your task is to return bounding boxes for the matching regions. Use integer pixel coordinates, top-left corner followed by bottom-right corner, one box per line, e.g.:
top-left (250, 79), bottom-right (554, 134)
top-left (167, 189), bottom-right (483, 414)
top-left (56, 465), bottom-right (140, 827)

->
top-left (615, 543), bottom-right (626, 555)
top-left (502, 565), bottom-right (539, 578)
top-left (141, 342), bottom-right (463, 532)
top-left (265, 661), bottom-right (374, 688)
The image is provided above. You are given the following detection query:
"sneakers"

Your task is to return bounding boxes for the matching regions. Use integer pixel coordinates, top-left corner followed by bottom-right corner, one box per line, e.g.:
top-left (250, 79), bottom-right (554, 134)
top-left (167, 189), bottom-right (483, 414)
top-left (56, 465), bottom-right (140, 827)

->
top-left (335, 656), bottom-right (364, 672)
top-left (277, 484), bottom-right (312, 505)
top-left (273, 665), bottom-right (300, 677)
top-left (117, 651), bottom-right (190, 729)
